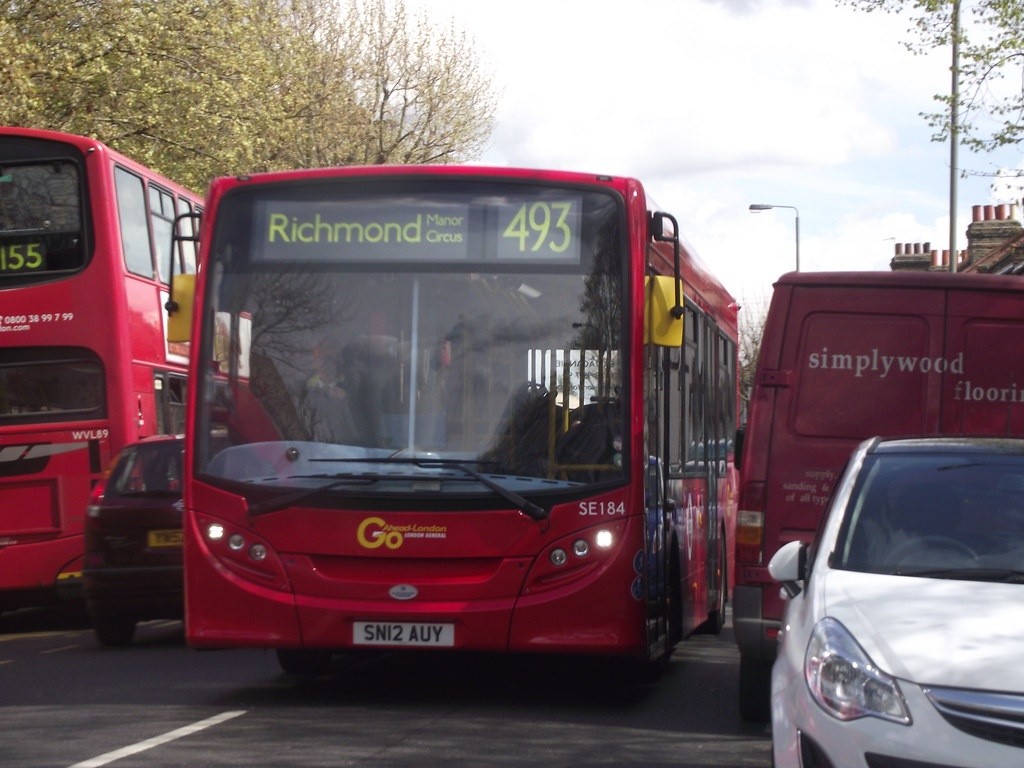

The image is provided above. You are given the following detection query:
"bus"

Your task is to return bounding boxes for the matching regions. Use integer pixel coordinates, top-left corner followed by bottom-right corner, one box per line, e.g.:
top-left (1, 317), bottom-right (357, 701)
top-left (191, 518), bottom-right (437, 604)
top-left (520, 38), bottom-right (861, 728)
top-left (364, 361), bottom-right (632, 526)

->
top-left (163, 165), bottom-right (740, 672)
top-left (0, 126), bottom-right (297, 594)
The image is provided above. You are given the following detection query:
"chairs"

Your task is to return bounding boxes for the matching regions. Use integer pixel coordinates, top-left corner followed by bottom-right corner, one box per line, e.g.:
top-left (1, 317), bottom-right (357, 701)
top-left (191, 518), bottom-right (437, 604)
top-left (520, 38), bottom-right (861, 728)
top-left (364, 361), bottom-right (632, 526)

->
top-left (345, 334), bottom-right (620, 479)
top-left (889, 487), bottom-right (996, 554)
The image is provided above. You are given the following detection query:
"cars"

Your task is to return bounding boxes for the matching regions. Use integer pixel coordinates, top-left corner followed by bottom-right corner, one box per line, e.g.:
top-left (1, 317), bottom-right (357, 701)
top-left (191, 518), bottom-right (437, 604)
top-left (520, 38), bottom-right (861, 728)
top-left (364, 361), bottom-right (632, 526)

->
top-left (677, 439), bottom-right (733, 458)
top-left (673, 460), bottom-right (728, 478)
top-left (644, 452), bottom-right (679, 573)
top-left (767, 435), bottom-right (1024, 768)
top-left (82, 431), bottom-right (232, 649)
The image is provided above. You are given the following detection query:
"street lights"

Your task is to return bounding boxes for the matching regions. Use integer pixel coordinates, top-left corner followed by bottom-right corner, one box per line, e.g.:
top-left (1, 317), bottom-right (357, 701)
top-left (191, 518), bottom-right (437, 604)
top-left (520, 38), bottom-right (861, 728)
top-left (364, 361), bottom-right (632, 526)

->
top-left (750, 203), bottom-right (800, 272)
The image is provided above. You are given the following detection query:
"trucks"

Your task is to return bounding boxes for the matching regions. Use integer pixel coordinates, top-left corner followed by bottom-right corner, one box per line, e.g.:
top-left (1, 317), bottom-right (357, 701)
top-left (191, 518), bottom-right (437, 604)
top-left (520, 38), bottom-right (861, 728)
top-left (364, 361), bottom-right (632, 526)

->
top-left (733, 272), bottom-right (1024, 721)
